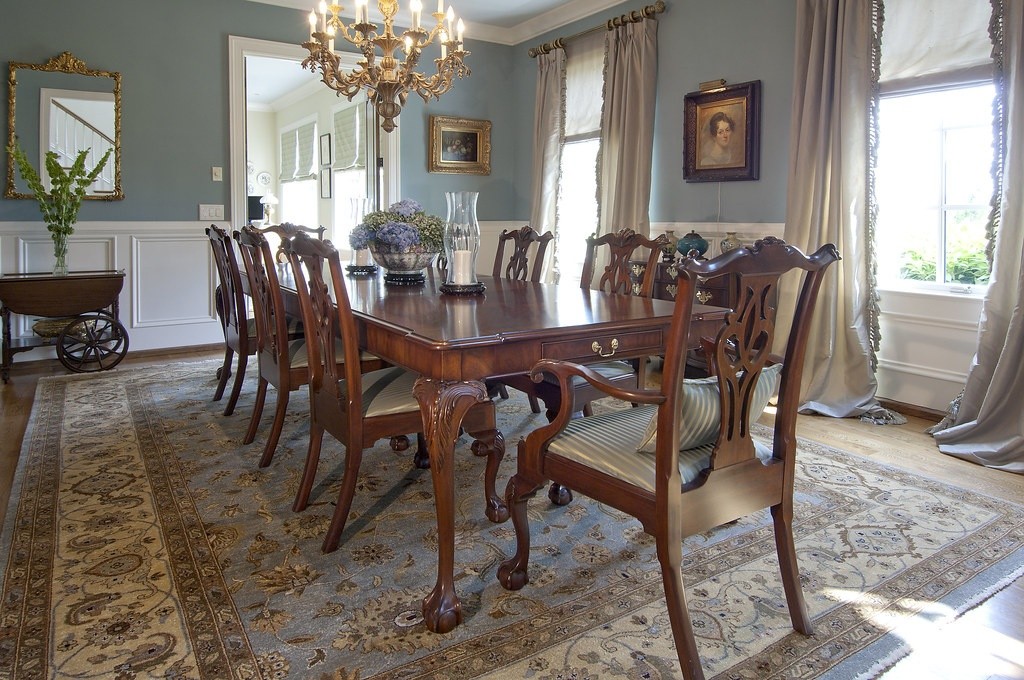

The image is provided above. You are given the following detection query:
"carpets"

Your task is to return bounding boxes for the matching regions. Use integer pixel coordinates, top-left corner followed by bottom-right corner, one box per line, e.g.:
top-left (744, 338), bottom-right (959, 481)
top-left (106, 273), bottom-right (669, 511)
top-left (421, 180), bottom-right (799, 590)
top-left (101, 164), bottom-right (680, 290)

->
top-left (0, 357), bottom-right (1024, 680)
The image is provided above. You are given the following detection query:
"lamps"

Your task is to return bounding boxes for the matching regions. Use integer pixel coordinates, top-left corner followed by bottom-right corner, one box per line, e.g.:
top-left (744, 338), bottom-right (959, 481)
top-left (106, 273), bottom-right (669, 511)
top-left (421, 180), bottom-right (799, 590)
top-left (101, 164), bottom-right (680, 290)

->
top-left (259, 194), bottom-right (278, 225)
top-left (699, 79), bottom-right (727, 90)
top-left (300, 0), bottom-right (471, 133)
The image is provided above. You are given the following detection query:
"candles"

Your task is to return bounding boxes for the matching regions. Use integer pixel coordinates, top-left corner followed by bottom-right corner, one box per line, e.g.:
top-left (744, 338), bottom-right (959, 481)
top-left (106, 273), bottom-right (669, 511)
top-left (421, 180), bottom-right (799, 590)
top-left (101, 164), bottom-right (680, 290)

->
top-left (454, 250), bottom-right (472, 283)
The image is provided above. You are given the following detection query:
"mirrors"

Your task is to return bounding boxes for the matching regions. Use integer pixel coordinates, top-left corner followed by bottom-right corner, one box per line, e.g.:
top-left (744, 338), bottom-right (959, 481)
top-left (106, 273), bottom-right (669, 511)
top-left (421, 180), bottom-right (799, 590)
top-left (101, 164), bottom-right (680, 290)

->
top-left (4, 51), bottom-right (125, 201)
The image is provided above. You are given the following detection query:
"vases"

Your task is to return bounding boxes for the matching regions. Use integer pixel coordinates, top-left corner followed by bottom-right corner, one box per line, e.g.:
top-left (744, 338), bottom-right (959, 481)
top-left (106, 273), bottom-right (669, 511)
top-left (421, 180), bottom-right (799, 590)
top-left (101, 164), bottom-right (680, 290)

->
top-left (356, 249), bottom-right (373, 267)
top-left (368, 241), bottom-right (439, 285)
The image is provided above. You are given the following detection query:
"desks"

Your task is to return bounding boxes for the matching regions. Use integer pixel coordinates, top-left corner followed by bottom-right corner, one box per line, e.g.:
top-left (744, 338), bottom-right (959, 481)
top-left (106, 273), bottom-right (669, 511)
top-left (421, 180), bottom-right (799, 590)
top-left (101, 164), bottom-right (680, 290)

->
top-left (236, 260), bottom-right (733, 633)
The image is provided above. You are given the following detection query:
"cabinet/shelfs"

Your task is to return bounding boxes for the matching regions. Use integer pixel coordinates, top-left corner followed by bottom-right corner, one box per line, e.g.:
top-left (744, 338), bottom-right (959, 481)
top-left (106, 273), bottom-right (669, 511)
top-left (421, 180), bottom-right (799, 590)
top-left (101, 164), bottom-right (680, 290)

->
top-left (624, 259), bottom-right (776, 376)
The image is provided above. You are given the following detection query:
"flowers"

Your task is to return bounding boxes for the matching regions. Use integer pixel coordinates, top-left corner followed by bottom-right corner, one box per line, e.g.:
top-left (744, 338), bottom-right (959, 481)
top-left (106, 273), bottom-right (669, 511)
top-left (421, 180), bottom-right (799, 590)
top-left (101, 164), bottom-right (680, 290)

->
top-left (349, 199), bottom-right (445, 253)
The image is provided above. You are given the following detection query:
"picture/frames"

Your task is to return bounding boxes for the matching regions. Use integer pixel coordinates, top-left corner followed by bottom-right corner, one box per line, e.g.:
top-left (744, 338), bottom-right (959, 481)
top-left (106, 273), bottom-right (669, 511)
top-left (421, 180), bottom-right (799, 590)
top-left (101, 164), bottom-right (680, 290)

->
top-left (320, 133), bottom-right (331, 165)
top-left (428, 114), bottom-right (492, 176)
top-left (320, 168), bottom-right (331, 198)
top-left (682, 80), bottom-right (759, 184)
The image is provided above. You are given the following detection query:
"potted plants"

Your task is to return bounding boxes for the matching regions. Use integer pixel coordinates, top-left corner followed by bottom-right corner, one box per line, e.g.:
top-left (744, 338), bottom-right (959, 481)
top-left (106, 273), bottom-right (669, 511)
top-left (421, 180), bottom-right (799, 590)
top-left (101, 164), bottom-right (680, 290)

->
top-left (6, 142), bottom-right (114, 276)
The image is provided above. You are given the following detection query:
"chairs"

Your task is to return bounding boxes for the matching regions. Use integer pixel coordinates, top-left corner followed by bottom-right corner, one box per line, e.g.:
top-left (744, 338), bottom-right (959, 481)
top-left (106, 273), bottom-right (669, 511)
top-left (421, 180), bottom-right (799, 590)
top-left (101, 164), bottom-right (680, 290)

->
top-left (204, 222), bottom-right (842, 680)
